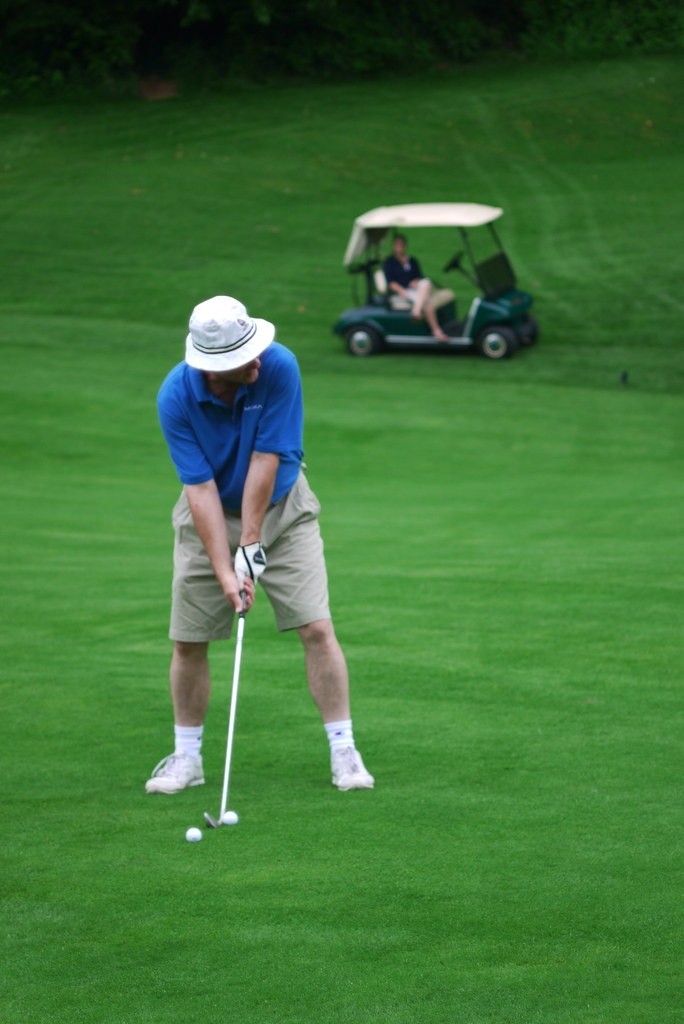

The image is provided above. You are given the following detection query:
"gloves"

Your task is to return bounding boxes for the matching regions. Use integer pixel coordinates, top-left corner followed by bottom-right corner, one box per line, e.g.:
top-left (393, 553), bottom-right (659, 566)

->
top-left (233, 541), bottom-right (266, 592)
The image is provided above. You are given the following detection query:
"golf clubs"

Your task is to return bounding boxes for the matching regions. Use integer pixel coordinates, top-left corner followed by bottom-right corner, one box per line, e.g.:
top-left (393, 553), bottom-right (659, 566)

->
top-left (202, 587), bottom-right (248, 828)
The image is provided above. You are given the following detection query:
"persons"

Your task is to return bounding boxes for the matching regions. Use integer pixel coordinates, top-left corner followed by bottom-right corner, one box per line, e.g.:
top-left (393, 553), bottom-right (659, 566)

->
top-left (145, 295), bottom-right (375, 795)
top-left (384, 233), bottom-right (455, 342)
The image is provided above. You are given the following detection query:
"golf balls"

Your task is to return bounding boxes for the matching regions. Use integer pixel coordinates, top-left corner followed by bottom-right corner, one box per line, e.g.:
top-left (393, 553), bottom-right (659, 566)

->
top-left (186, 827), bottom-right (202, 842)
top-left (222, 811), bottom-right (239, 825)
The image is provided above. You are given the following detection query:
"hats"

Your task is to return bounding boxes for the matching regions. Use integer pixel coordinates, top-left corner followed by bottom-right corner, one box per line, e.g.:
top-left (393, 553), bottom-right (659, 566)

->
top-left (185, 296), bottom-right (275, 371)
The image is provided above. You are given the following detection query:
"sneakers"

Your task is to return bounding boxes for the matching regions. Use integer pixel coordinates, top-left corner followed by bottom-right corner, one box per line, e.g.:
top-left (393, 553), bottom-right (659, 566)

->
top-left (145, 751), bottom-right (205, 795)
top-left (330, 746), bottom-right (374, 791)
